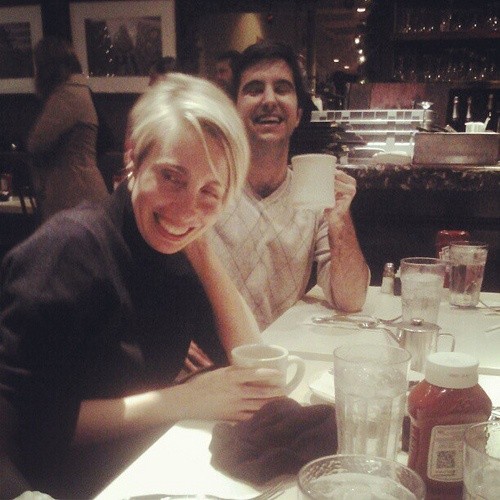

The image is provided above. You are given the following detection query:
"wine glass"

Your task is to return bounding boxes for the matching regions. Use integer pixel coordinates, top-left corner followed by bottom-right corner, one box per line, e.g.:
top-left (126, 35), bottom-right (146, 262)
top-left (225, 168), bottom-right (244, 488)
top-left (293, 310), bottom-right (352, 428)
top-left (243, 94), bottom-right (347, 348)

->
top-left (393, 5), bottom-right (500, 85)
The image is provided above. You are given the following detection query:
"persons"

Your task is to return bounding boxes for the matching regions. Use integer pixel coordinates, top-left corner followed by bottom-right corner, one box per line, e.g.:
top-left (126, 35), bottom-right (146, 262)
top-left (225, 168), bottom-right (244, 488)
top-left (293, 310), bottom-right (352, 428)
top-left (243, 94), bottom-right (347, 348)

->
top-left (29, 36), bottom-right (111, 224)
top-left (148, 56), bottom-right (179, 84)
top-left (182, 44), bottom-right (367, 379)
top-left (0, 73), bottom-right (293, 500)
top-left (217, 50), bottom-right (239, 87)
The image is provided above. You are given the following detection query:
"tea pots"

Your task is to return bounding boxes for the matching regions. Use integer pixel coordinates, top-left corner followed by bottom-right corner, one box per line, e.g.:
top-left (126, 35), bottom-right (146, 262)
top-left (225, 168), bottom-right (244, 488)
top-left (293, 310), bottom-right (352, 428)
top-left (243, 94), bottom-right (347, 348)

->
top-left (384, 318), bottom-right (454, 372)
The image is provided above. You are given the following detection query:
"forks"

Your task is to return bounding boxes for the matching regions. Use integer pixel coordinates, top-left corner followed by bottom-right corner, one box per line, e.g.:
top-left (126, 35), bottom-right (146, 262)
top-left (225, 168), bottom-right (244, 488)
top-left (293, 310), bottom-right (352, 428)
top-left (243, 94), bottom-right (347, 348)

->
top-left (315, 312), bottom-right (401, 323)
top-left (128, 479), bottom-right (288, 500)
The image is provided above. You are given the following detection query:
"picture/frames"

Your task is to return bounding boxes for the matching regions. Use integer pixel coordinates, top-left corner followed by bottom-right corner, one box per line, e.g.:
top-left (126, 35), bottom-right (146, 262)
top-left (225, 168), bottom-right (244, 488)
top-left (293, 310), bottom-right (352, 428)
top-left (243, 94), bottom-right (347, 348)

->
top-left (65, 0), bottom-right (178, 96)
top-left (0, 4), bottom-right (50, 97)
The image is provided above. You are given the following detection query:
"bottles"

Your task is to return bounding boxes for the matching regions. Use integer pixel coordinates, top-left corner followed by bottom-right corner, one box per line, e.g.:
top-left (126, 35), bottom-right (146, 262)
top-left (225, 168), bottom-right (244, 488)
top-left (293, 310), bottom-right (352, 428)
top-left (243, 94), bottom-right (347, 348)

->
top-left (435, 229), bottom-right (469, 301)
top-left (451, 93), bottom-right (495, 131)
top-left (392, 263), bottom-right (402, 296)
top-left (407, 352), bottom-right (492, 500)
top-left (380, 261), bottom-right (394, 295)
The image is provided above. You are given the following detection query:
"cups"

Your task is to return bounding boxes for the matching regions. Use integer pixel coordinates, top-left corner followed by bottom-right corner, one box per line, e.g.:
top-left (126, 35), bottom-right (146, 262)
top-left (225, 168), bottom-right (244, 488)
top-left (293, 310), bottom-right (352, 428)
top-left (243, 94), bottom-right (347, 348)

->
top-left (463, 421), bottom-right (500, 499)
top-left (448, 241), bottom-right (488, 307)
top-left (230, 344), bottom-right (305, 399)
top-left (399, 257), bottom-right (446, 324)
top-left (333, 341), bottom-right (412, 463)
top-left (297, 454), bottom-right (427, 500)
top-left (291, 154), bottom-right (337, 210)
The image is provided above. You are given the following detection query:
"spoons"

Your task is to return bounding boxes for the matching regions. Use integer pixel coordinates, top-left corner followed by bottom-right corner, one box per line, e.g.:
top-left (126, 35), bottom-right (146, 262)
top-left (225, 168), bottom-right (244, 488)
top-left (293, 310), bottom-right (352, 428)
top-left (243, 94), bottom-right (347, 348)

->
top-left (311, 314), bottom-right (380, 329)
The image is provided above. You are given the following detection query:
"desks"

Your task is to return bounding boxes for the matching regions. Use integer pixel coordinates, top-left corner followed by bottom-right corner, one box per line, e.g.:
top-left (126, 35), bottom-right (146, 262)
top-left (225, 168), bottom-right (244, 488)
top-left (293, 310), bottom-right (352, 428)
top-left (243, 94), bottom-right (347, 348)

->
top-left (90, 285), bottom-right (499, 499)
